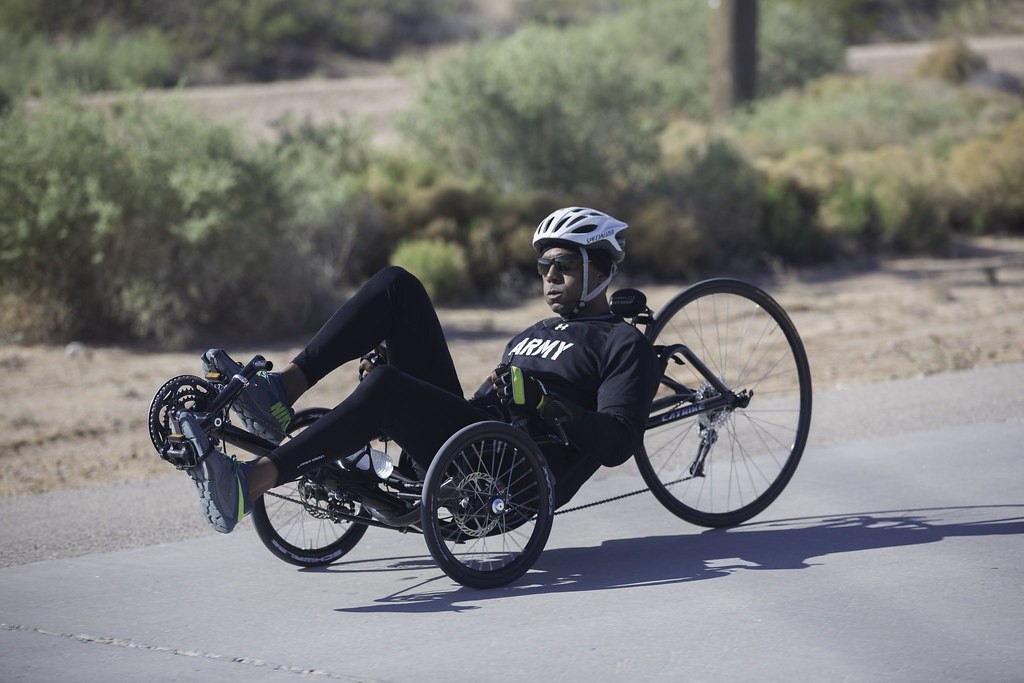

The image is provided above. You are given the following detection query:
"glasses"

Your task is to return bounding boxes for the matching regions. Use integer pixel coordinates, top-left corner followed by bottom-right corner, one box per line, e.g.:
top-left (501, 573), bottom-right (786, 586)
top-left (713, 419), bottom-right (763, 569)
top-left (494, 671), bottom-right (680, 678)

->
top-left (537, 253), bottom-right (593, 275)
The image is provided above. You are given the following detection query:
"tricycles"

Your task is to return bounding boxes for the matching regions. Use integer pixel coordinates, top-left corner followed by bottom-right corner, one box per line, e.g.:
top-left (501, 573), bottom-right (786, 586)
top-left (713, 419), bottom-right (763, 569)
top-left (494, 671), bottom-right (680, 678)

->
top-left (149, 278), bottom-right (812, 594)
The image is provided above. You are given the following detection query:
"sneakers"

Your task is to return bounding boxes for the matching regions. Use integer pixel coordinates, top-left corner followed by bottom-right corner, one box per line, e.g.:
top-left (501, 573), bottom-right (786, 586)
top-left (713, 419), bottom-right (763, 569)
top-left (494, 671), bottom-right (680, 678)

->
top-left (178, 411), bottom-right (253, 534)
top-left (204, 349), bottom-right (294, 446)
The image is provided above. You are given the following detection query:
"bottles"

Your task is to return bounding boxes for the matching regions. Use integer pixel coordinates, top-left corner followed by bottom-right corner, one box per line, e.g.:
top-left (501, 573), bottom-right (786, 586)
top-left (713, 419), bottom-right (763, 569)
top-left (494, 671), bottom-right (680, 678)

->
top-left (350, 448), bottom-right (396, 480)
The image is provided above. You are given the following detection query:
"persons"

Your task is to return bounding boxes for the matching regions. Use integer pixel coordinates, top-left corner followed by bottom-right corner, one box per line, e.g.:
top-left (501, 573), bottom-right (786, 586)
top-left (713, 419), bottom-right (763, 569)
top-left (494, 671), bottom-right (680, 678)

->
top-left (175, 207), bottom-right (660, 536)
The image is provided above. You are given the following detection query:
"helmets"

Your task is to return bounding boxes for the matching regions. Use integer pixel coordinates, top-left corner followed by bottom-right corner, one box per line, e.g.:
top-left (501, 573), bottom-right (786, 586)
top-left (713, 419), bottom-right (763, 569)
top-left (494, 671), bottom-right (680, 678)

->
top-left (532, 206), bottom-right (629, 264)
